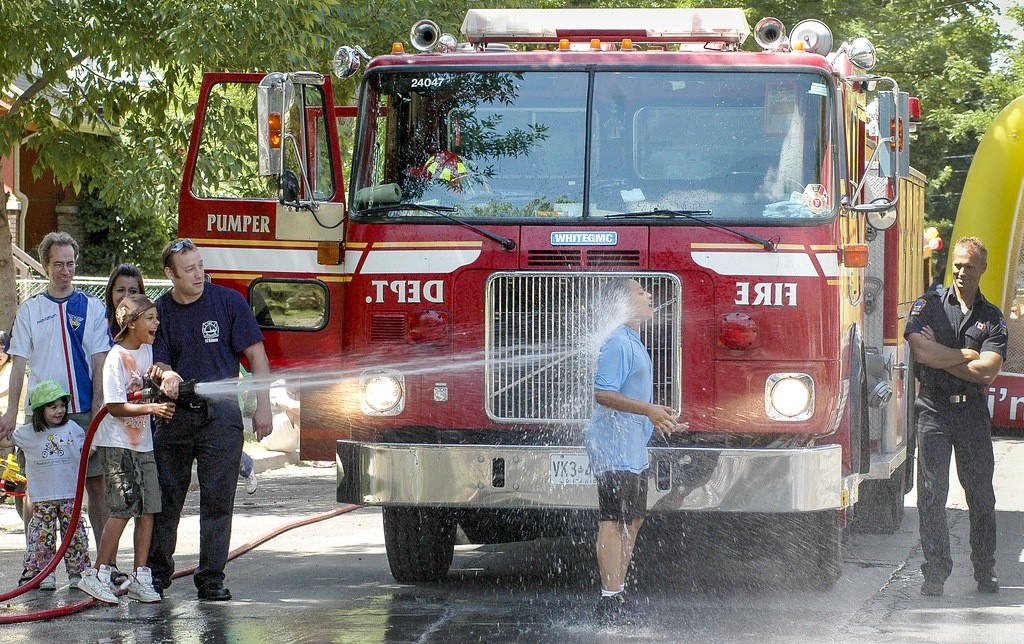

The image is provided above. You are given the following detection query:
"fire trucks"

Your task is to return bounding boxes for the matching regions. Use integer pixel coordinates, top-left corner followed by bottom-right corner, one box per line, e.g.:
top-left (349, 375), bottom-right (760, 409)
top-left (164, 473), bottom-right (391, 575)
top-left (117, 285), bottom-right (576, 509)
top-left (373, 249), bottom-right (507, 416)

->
top-left (179, 7), bottom-right (943, 582)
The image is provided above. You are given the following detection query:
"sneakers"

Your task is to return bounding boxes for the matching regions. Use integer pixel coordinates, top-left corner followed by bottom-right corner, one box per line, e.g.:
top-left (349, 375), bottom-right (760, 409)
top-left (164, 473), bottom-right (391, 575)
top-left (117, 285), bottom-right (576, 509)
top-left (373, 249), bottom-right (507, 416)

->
top-left (41, 570), bottom-right (56, 590)
top-left (595, 593), bottom-right (652, 631)
top-left (77, 564), bottom-right (119, 604)
top-left (128, 566), bottom-right (161, 602)
top-left (245, 468), bottom-right (257, 494)
top-left (69, 571), bottom-right (82, 588)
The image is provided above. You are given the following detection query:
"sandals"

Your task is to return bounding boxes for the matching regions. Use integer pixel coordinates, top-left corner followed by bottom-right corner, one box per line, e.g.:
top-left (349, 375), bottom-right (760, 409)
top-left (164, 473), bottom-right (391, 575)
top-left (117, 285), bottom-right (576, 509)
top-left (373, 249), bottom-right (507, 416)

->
top-left (17, 569), bottom-right (39, 588)
top-left (108, 564), bottom-right (129, 584)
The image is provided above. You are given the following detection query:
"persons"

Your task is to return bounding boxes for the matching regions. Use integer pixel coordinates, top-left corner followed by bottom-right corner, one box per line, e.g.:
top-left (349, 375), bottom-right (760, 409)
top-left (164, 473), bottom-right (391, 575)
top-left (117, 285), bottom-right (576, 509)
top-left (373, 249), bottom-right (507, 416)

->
top-left (0, 232), bottom-right (176, 604)
top-left (239, 451), bottom-right (258, 493)
top-left (142, 241), bottom-right (274, 599)
top-left (585, 278), bottom-right (690, 626)
top-left (903, 236), bottom-right (1008, 595)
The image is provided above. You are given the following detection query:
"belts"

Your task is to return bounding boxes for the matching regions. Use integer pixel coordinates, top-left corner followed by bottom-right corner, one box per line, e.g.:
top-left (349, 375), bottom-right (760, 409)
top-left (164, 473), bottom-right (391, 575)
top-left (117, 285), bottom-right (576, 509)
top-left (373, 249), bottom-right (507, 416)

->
top-left (175, 401), bottom-right (207, 412)
top-left (938, 393), bottom-right (974, 406)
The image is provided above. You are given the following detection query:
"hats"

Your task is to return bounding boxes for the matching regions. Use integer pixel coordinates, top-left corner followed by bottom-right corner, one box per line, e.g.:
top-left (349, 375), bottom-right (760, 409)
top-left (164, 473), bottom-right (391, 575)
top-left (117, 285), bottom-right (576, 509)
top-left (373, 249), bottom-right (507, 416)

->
top-left (28, 381), bottom-right (72, 411)
top-left (111, 293), bottom-right (156, 342)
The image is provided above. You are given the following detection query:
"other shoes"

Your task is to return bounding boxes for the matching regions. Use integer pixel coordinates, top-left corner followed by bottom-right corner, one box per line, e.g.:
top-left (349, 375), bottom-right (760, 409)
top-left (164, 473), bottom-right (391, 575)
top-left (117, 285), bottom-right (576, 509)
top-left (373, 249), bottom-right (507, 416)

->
top-left (920, 580), bottom-right (944, 597)
top-left (977, 576), bottom-right (999, 593)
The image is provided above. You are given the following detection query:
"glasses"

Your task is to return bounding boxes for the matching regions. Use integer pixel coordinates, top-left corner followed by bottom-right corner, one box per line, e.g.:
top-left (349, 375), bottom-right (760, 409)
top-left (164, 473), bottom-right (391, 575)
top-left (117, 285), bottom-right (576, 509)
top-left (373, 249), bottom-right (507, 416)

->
top-left (164, 239), bottom-right (193, 265)
top-left (49, 263), bottom-right (79, 269)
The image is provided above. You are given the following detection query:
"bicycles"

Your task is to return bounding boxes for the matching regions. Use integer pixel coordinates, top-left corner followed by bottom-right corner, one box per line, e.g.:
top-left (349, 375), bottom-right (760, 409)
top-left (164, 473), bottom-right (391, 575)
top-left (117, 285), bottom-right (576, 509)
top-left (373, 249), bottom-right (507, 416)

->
top-left (0, 454), bottom-right (26, 520)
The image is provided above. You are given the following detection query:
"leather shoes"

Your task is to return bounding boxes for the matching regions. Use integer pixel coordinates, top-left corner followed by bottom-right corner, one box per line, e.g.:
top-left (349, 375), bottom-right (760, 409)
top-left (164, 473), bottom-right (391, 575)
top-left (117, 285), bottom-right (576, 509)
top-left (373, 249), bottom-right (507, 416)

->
top-left (198, 585), bottom-right (231, 600)
top-left (155, 582), bottom-right (164, 600)
